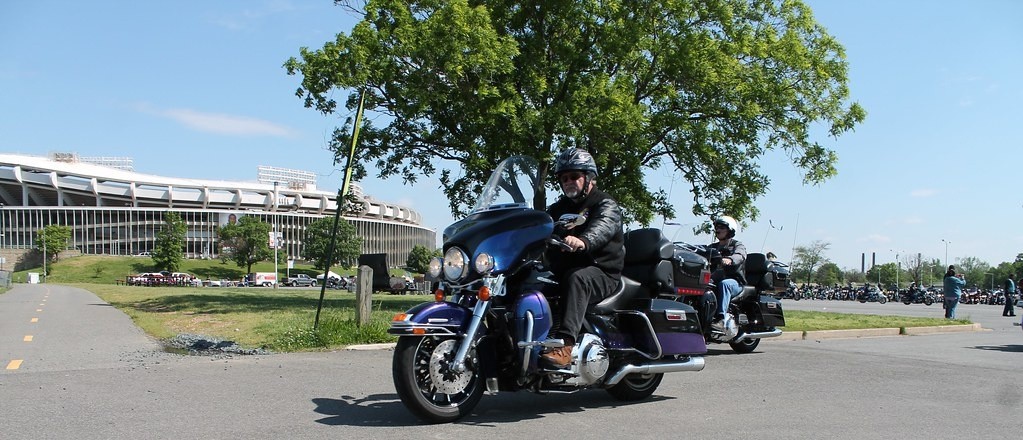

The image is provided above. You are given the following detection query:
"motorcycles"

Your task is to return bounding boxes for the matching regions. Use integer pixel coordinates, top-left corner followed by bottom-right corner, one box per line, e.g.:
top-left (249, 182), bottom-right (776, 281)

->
top-left (781, 276), bottom-right (1023, 304)
top-left (655, 225), bottom-right (794, 355)
top-left (387, 152), bottom-right (712, 420)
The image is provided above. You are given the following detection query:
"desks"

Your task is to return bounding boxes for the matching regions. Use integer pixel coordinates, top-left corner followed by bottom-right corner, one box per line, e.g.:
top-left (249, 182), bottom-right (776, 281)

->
top-left (126, 275), bottom-right (197, 288)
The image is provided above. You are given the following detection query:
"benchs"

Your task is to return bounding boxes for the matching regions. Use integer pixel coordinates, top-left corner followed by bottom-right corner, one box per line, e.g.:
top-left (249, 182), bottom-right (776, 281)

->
top-left (115, 279), bottom-right (192, 285)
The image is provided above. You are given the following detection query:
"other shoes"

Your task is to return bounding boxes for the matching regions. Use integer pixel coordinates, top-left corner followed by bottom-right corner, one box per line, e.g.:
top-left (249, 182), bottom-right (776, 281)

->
top-left (1003, 314), bottom-right (1009, 316)
top-left (1011, 313), bottom-right (1016, 316)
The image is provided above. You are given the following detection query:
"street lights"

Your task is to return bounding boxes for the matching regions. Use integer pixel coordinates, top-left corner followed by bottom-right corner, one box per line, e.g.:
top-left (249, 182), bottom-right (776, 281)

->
top-left (889, 249), bottom-right (906, 295)
top-left (941, 237), bottom-right (952, 278)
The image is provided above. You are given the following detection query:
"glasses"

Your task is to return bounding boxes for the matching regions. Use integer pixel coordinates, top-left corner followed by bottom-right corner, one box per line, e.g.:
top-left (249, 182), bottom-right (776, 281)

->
top-left (561, 173), bottom-right (586, 183)
top-left (715, 226), bottom-right (727, 230)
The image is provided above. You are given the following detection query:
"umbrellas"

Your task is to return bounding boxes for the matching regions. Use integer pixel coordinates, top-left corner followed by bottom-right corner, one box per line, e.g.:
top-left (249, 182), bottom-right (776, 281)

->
top-left (125, 271), bottom-right (190, 282)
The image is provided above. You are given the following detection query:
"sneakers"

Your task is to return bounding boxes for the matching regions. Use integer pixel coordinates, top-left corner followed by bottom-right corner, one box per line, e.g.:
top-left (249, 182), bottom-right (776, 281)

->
top-left (710, 321), bottom-right (725, 332)
top-left (542, 346), bottom-right (572, 370)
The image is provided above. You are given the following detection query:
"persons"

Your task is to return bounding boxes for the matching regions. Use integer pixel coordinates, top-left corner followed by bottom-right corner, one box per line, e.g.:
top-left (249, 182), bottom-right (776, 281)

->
top-left (1019, 278), bottom-right (1023, 331)
top-left (910, 282), bottom-right (920, 300)
top-left (189, 274), bottom-right (197, 287)
top-left (943, 265), bottom-right (966, 320)
top-left (864, 283), bottom-right (875, 299)
top-left (542, 148), bottom-right (624, 369)
top-left (229, 214), bottom-right (236, 225)
top-left (708, 216), bottom-right (747, 332)
top-left (1003, 274), bottom-right (1016, 316)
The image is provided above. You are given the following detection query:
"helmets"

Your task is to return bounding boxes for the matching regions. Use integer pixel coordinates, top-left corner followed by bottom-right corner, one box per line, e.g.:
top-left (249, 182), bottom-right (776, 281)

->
top-left (555, 148), bottom-right (598, 177)
top-left (714, 216), bottom-right (737, 237)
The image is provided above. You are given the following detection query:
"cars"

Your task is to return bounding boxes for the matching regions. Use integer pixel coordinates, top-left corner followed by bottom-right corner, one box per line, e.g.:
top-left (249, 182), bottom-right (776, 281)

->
top-left (129, 271), bottom-right (356, 293)
